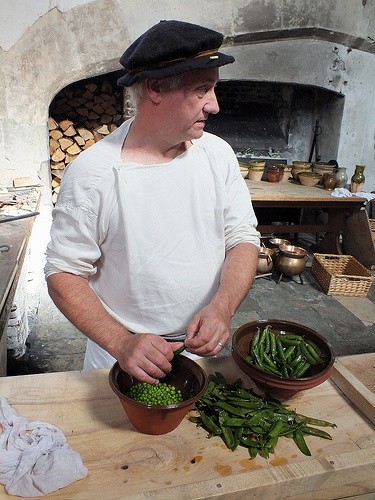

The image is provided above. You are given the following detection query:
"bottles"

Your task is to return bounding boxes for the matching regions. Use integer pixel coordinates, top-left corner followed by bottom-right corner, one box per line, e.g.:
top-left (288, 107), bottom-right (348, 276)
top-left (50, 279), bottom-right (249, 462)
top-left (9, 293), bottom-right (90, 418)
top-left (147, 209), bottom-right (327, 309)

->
top-left (323, 172), bottom-right (336, 190)
top-left (336, 167), bottom-right (348, 188)
top-left (351, 164), bottom-right (366, 194)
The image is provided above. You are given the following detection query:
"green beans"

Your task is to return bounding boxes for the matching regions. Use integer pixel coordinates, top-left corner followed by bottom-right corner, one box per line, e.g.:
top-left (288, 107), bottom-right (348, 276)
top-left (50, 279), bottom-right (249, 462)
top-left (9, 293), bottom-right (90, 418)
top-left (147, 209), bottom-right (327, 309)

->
top-left (173, 344), bottom-right (186, 356)
top-left (243, 325), bottom-right (324, 378)
top-left (125, 381), bottom-right (184, 405)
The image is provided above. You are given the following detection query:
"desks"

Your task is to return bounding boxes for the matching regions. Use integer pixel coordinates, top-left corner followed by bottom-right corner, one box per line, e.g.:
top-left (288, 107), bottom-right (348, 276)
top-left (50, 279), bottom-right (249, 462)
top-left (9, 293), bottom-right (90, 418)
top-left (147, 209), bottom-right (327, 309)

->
top-left (0, 352), bottom-right (375, 500)
top-left (244, 177), bottom-right (367, 262)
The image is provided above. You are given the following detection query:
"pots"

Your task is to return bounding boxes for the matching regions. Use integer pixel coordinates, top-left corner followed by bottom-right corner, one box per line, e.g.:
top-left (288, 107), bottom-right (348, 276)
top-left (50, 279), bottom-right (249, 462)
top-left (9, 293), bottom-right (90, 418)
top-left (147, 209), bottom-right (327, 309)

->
top-left (256, 238), bottom-right (309, 285)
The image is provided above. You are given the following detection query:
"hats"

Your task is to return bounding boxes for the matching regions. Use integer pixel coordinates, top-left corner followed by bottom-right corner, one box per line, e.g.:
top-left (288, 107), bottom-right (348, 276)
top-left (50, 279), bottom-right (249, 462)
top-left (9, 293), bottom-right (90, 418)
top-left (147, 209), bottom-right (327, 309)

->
top-left (117, 19), bottom-right (235, 87)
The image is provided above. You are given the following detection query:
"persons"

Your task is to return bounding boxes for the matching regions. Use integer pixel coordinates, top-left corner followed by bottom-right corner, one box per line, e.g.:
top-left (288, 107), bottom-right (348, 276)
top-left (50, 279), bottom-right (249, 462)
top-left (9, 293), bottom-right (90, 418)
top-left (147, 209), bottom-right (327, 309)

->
top-left (43, 20), bottom-right (261, 387)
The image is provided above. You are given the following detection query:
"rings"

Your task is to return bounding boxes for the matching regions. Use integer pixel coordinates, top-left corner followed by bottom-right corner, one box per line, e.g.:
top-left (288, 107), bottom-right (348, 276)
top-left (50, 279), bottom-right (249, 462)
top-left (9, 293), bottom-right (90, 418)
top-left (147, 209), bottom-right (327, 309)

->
top-left (218, 343), bottom-right (224, 350)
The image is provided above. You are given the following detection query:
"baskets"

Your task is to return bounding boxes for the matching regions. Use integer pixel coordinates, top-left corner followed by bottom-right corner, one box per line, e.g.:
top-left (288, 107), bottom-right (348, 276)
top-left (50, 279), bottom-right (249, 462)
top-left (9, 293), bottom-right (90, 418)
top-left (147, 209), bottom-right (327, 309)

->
top-left (311, 253), bottom-right (374, 297)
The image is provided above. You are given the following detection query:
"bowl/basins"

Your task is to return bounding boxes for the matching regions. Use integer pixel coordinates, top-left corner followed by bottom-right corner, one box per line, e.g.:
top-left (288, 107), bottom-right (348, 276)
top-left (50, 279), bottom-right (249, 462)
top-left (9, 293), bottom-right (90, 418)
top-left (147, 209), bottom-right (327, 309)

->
top-left (109, 354), bottom-right (210, 436)
top-left (231, 319), bottom-right (336, 401)
top-left (238, 159), bottom-right (337, 187)
